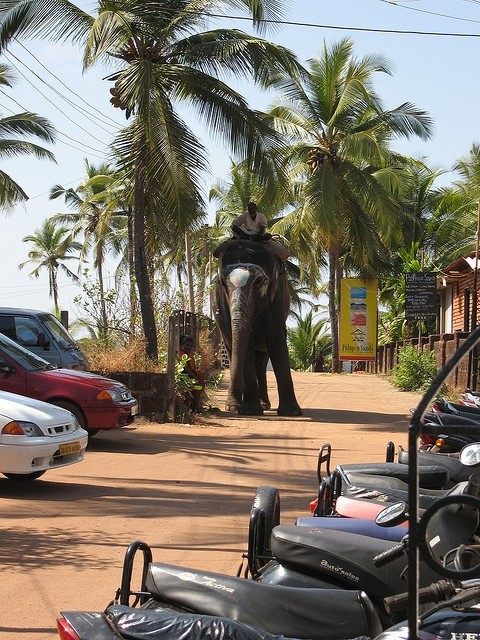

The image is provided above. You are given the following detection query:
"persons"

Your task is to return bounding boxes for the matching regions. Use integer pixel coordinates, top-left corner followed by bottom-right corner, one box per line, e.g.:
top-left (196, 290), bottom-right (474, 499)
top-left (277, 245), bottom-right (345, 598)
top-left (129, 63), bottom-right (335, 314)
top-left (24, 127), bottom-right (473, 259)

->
top-left (176, 337), bottom-right (205, 414)
top-left (230, 201), bottom-right (273, 243)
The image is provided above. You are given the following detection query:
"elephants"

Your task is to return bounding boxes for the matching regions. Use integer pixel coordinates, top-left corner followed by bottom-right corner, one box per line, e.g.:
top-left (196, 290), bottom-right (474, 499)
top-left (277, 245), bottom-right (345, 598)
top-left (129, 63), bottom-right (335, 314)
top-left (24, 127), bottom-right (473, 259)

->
top-left (209, 237), bottom-right (303, 416)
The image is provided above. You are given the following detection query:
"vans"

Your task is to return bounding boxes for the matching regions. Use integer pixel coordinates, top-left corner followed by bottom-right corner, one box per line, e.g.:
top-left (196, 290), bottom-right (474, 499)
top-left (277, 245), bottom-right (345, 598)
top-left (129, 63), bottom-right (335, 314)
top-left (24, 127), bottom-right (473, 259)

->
top-left (0, 307), bottom-right (89, 370)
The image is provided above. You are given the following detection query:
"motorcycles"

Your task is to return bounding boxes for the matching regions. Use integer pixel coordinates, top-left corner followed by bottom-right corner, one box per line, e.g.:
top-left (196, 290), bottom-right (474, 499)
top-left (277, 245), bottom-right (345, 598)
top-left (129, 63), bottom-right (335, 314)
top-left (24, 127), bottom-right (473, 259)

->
top-left (56, 387), bottom-right (480, 640)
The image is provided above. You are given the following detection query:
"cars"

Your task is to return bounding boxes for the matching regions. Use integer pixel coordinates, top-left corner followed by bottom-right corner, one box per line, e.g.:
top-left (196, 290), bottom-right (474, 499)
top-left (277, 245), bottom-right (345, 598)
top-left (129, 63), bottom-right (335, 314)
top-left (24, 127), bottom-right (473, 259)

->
top-left (0, 390), bottom-right (89, 480)
top-left (0, 334), bottom-right (139, 437)
